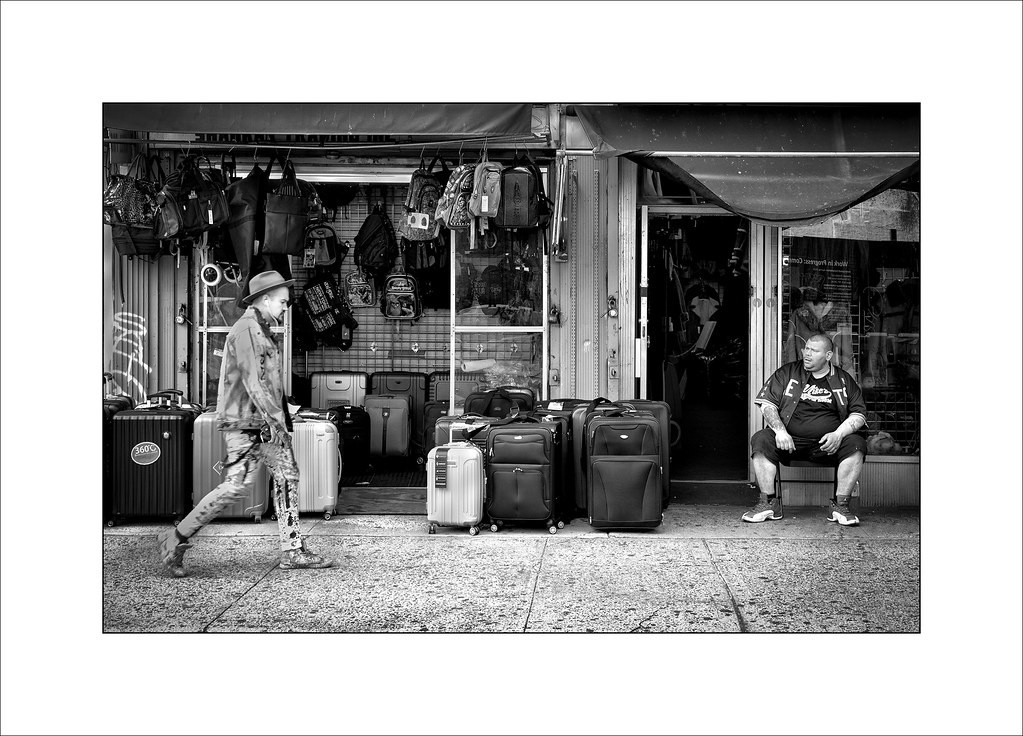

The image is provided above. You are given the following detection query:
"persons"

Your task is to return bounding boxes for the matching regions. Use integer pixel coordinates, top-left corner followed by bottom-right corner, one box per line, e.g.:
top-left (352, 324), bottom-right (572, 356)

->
top-left (741, 334), bottom-right (871, 526)
top-left (157, 270), bottom-right (334, 577)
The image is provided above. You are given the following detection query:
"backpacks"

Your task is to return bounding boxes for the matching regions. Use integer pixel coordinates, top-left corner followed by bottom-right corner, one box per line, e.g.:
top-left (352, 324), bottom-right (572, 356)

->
top-left (398, 159), bottom-right (443, 241)
top-left (399, 235), bottom-right (448, 310)
top-left (355, 203), bottom-right (399, 276)
top-left (455, 251), bottom-right (543, 326)
top-left (468, 147), bottom-right (552, 253)
top-left (380, 272), bottom-right (423, 320)
top-left (434, 157), bottom-right (478, 231)
top-left (344, 270), bottom-right (377, 307)
top-left (302, 220), bottom-right (350, 270)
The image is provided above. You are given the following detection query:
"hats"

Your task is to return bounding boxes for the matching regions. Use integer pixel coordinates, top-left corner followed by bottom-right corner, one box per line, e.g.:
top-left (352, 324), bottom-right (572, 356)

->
top-left (242, 270), bottom-right (296, 303)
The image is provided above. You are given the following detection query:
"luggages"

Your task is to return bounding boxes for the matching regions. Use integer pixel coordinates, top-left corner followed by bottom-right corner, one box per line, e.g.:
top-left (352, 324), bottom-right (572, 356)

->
top-left (102, 369), bottom-right (671, 536)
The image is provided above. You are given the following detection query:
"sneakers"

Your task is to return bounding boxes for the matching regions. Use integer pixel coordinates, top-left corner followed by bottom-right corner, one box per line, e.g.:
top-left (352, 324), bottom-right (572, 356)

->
top-left (742, 493), bottom-right (783, 523)
top-left (157, 528), bottom-right (194, 577)
top-left (279, 540), bottom-right (333, 569)
top-left (826, 494), bottom-right (859, 525)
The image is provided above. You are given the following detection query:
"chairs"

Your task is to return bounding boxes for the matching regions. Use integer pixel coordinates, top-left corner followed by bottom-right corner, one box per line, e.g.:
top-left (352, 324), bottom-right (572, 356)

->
top-left (762, 415), bottom-right (842, 517)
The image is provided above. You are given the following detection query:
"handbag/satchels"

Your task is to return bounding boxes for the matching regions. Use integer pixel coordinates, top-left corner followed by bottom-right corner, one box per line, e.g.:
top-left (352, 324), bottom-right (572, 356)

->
top-left (263, 159), bottom-right (321, 259)
top-left (103, 151), bottom-right (243, 255)
top-left (304, 273), bottom-right (347, 332)
